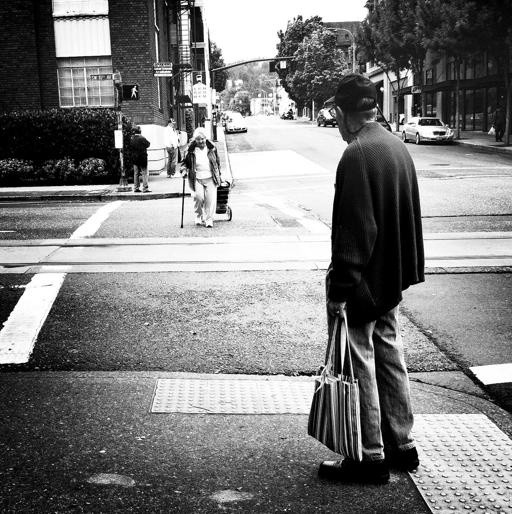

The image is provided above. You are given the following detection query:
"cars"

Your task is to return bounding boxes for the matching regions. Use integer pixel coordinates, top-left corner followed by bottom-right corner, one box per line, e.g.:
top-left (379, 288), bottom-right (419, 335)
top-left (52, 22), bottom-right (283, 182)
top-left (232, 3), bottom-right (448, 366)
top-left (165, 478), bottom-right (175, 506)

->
top-left (417, 102), bottom-right (438, 116)
top-left (375, 115), bottom-right (390, 132)
top-left (399, 112), bottom-right (405, 123)
top-left (318, 107), bottom-right (339, 128)
top-left (221, 108), bottom-right (249, 132)
top-left (469, 107), bottom-right (502, 132)
top-left (400, 117), bottom-right (457, 144)
top-left (280, 111), bottom-right (295, 120)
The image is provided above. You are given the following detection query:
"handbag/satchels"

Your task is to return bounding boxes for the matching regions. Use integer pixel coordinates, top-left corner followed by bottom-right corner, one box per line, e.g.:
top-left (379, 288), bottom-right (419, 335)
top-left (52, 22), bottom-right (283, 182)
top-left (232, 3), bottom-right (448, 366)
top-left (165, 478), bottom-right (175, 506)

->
top-left (306, 310), bottom-right (363, 463)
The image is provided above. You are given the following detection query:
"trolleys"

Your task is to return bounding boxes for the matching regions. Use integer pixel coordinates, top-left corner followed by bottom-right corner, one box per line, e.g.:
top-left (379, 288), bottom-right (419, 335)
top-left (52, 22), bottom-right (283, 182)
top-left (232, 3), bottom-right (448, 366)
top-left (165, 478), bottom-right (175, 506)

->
top-left (212, 180), bottom-right (234, 222)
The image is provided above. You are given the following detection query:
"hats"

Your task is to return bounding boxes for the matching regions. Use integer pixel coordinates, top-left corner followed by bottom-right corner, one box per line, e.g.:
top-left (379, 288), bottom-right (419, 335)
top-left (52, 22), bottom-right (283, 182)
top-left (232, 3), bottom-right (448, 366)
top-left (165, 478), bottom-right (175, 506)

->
top-left (322, 74), bottom-right (378, 111)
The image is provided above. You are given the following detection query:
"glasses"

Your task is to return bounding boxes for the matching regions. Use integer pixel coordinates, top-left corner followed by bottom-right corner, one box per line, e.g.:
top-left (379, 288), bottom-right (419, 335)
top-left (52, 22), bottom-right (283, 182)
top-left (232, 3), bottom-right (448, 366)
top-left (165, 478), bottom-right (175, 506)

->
top-left (330, 103), bottom-right (343, 119)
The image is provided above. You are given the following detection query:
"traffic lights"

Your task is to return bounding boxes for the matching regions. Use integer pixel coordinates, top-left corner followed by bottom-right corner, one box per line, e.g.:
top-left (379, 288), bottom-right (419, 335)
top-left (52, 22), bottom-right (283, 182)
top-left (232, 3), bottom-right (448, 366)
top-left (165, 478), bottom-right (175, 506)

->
top-left (122, 84), bottom-right (140, 100)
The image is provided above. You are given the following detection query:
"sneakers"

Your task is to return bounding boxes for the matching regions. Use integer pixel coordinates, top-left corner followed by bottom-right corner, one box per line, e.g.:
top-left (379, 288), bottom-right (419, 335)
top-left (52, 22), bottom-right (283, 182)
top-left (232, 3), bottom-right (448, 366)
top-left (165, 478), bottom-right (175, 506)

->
top-left (134, 187), bottom-right (152, 193)
top-left (196, 216), bottom-right (212, 227)
top-left (379, 449), bottom-right (422, 472)
top-left (319, 453), bottom-right (391, 483)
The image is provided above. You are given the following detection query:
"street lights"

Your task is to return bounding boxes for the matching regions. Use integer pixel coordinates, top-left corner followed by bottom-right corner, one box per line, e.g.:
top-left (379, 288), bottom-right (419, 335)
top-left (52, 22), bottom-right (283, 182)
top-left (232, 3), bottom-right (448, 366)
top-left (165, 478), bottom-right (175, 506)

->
top-left (319, 23), bottom-right (358, 78)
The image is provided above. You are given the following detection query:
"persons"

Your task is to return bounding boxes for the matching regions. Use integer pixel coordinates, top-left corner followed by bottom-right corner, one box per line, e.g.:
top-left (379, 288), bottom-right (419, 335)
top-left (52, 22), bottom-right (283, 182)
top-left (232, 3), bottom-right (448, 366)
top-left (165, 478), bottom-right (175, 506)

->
top-left (179, 127), bottom-right (224, 228)
top-left (318, 74), bottom-right (425, 485)
top-left (492, 105), bottom-right (505, 142)
top-left (129, 126), bottom-right (152, 192)
top-left (163, 119), bottom-right (177, 178)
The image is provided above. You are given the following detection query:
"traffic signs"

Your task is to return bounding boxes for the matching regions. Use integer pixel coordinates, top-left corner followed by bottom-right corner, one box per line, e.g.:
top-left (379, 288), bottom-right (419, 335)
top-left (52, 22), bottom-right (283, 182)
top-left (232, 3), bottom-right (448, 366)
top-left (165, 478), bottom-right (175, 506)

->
top-left (89, 73), bottom-right (113, 81)
